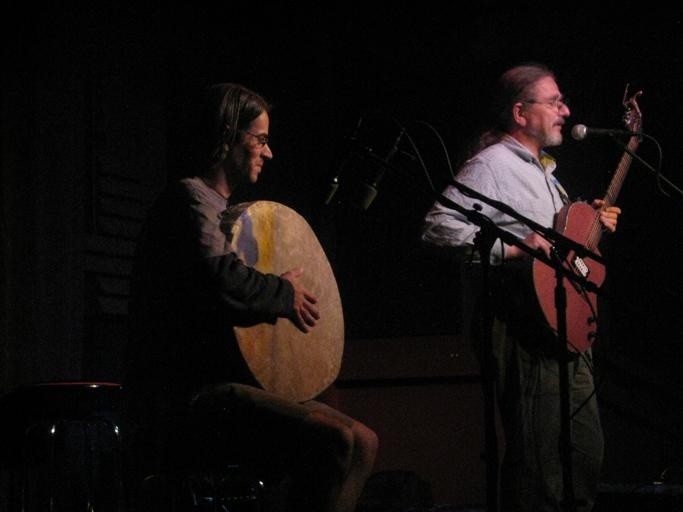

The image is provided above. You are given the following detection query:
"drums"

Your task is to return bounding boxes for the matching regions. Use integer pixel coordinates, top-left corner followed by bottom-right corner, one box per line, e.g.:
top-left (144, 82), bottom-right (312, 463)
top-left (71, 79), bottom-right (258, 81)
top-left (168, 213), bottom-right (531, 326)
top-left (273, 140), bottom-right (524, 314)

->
top-left (219, 200), bottom-right (344, 405)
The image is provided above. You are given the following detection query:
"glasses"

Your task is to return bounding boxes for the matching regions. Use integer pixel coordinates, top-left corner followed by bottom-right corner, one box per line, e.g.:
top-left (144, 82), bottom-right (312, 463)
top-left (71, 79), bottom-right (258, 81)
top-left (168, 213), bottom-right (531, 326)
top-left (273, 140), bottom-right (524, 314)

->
top-left (243, 130), bottom-right (270, 144)
top-left (524, 98), bottom-right (560, 110)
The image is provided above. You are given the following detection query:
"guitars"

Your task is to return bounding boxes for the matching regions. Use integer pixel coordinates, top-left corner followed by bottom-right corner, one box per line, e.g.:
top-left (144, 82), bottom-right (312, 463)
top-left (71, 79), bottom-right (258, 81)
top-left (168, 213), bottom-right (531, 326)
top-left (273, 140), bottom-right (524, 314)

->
top-left (498, 84), bottom-right (642, 365)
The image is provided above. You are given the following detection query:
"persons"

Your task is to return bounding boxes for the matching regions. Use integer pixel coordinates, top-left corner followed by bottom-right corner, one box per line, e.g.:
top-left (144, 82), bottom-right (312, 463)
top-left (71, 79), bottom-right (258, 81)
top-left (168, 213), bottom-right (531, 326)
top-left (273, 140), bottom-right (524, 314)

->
top-left (416, 63), bottom-right (625, 510)
top-left (127, 82), bottom-right (380, 510)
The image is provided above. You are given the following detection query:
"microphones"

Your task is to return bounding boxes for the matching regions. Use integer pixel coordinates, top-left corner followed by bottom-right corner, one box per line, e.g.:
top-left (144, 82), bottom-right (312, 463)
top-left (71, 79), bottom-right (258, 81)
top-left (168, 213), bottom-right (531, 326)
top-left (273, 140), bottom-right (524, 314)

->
top-left (355, 138), bottom-right (398, 209)
top-left (570, 123), bottom-right (625, 140)
top-left (321, 120), bottom-right (361, 205)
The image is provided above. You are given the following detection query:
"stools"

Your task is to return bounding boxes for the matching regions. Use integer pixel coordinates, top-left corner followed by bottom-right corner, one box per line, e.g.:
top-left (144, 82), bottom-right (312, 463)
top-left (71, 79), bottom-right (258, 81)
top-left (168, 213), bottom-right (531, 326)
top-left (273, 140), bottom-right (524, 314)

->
top-left (30, 382), bottom-right (123, 512)
top-left (183, 403), bottom-right (266, 511)
